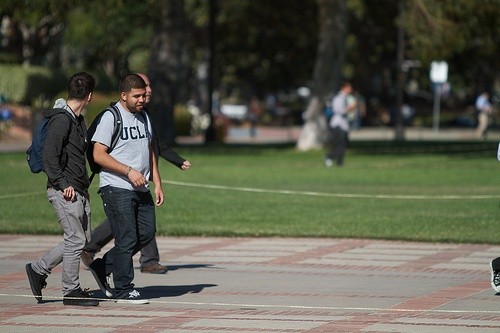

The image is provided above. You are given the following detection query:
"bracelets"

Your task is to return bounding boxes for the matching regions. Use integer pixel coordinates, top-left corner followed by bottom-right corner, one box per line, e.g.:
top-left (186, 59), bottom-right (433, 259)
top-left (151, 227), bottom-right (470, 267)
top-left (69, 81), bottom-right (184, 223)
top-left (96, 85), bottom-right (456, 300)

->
top-left (125, 167), bottom-right (132, 176)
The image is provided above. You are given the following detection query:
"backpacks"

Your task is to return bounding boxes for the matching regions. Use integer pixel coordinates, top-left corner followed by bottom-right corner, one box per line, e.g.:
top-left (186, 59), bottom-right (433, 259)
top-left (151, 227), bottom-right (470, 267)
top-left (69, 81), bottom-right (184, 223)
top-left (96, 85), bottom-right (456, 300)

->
top-left (86, 101), bottom-right (147, 187)
top-left (26, 114), bottom-right (73, 173)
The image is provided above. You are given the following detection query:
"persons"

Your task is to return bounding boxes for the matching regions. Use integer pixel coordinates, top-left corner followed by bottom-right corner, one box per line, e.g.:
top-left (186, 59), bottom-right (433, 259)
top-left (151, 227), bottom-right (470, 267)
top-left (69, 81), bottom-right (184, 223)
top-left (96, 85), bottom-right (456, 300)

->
top-left (88, 74), bottom-right (164, 304)
top-left (490, 256), bottom-right (500, 292)
top-left (81, 73), bottom-right (190, 274)
top-left (211, 82), bottom-right (492, 167)
top-left (26, 71), bottom-right (99, 307)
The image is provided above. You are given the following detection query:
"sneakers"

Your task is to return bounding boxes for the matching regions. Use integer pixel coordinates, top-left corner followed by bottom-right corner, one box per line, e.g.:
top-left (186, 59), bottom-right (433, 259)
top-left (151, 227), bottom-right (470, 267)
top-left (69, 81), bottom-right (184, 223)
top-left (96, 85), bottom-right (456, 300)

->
top-left (111, 287), bottom-right (150, 305)
top-left (25, 262), bottom-right (48, 303)
top-left (88, 258), bottom-right (114, 298)
top-left (63, 287), bottom-right (100, 306)
top-left (489, 258), bottom-right (500, 293)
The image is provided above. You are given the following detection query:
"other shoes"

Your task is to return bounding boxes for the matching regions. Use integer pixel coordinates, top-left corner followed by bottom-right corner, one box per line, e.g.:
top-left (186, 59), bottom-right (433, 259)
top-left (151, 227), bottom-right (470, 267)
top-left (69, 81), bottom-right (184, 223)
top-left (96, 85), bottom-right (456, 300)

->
top-left (81, 251), bottom-right (95, 269)
top-left (140, 264), bottom-right (169, 274)
